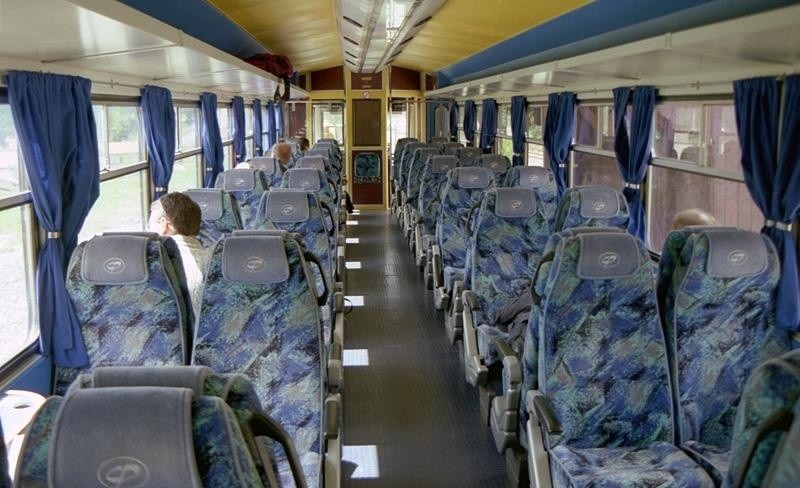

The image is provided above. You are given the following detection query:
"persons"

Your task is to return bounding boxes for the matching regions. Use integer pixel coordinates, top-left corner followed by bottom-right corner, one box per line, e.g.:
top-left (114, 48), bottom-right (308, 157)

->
top-left (288, 137), bottom-right (311, 153)
top-left (143, 190), bottom-right (215, 319)
top-left (266, 140), bottom-right (294, 169)
top-left (671, 207), bottom-right (726, 228)
